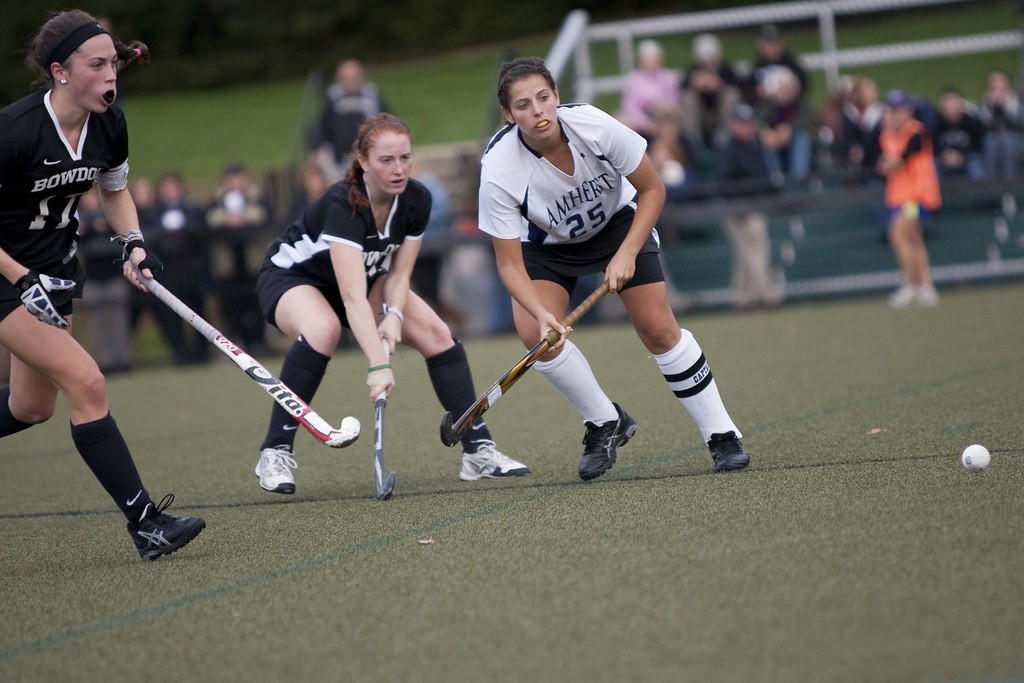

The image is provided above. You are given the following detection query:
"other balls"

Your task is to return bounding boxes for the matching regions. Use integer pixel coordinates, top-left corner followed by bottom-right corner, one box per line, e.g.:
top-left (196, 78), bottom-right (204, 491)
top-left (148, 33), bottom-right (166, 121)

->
top-left (961, 444), bottom-right (990, 473)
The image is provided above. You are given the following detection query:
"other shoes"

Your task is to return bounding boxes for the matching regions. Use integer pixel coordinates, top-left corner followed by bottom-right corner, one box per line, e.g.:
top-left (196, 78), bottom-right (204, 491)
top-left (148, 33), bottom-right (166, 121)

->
top-left (889, 284), bottom-right (941, 308)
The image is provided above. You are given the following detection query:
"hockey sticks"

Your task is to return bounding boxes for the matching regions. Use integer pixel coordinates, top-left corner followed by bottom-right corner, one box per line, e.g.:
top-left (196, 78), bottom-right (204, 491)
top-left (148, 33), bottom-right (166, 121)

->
top-left (439, 278), bottom-right (612, 449)
top-left (112, 256), bottom-right (362, 449)
top-left (372, 337), bottom-right (397, 502)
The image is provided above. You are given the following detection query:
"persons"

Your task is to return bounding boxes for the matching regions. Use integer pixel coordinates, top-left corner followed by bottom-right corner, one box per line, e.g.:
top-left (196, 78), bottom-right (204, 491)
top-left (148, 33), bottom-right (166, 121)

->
top-left (253, 113), bottom-right (530, 493)
top-left (288, 58), bottom-right (452, 307)
top-left (878, 90), bottom-right (943, 307)
top-left (622, 23), bottom-right (814, 194)
top-left (815, 70), bottom-right (1024, 182)
top-left (0, 10), bottom-right (206, 562)
top-left (72, 164), bottom-right (273, 371)
top-left (478, 56), bottom-right (750, 481)
top-left (709, 103), bottom-right (789, 306)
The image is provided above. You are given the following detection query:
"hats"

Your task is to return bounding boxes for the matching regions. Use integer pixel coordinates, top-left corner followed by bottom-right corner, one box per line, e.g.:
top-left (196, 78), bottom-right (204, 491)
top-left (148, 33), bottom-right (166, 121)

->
top-left (875, 89), bottom-right (915, 111)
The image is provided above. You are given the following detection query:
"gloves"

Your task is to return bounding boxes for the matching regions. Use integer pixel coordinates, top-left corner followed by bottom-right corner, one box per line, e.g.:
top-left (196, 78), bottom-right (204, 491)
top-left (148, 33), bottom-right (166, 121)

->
top-left (11, 269), bottom-right (77, 330)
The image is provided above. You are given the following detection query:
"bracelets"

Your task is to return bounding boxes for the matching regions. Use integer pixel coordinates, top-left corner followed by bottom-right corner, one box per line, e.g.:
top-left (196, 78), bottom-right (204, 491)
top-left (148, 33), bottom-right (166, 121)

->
top-left (110, 230), bottom-right (144, 246)
top-left (382, 304), bottom-right (403, 321)
top-left (368, 364), bottom-right (391, 372)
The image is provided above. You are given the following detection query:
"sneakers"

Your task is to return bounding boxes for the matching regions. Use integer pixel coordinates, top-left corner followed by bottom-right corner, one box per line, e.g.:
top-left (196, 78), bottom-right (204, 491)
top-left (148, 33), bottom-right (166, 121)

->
top-left (459, 439), bottom-right (531, 482)
top-left (127, 494), bottom-right (206, 563)
top-left (578, 402), bottom-right (638, 481)
top-left (707, 430), bottom-right (750, 471)
top-left (254, 444), bottom-right (298, 495)
